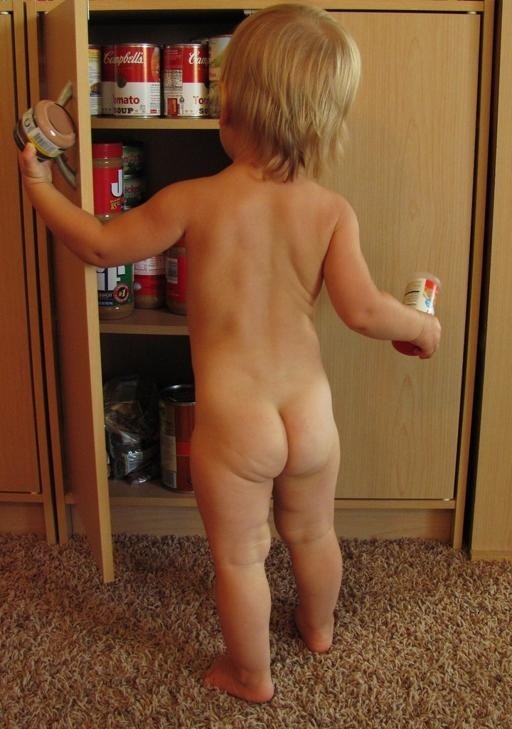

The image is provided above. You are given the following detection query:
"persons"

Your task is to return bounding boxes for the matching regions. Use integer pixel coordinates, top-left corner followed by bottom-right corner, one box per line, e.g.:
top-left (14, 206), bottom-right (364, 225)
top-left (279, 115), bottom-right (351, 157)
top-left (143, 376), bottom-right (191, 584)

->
top-left (17, 0), bottom-right (442, 706)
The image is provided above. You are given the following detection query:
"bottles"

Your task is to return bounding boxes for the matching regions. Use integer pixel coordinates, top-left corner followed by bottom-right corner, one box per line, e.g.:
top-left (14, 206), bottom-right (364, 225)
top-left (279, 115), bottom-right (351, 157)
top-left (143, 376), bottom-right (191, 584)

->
top-left (392, 269), bottom-right (442, 358)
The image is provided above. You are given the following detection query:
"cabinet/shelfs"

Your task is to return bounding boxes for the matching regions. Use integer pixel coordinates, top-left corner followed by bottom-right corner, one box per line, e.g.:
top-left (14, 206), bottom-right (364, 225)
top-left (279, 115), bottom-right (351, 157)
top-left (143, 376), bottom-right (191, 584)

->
top-left (0, 0), bottom-right (512, 585)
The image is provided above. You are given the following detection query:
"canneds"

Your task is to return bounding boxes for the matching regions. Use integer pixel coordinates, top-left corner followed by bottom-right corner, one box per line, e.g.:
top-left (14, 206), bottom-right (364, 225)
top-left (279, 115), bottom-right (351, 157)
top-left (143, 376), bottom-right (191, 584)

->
top-left (158, 384), bottom-right (196, 495)
top-left (13, 98), bottom-right (77, 163)
top-left (92, 142), bottom-right (186, 321)
top-left (88, 34), bottom-right (233, 119)
